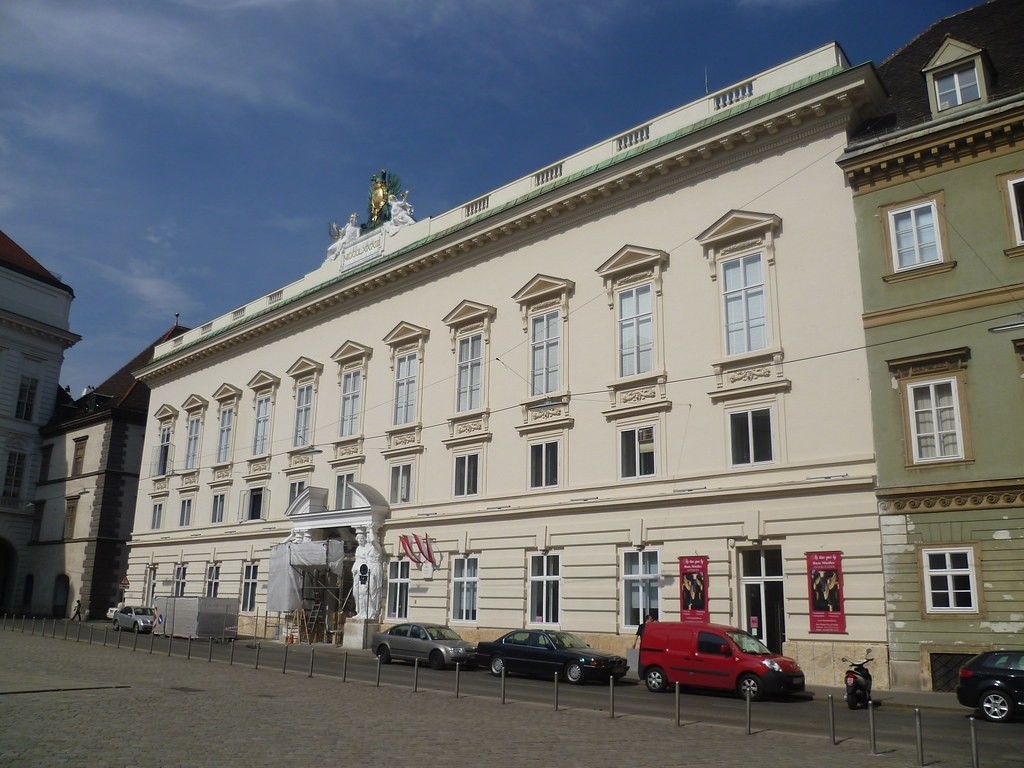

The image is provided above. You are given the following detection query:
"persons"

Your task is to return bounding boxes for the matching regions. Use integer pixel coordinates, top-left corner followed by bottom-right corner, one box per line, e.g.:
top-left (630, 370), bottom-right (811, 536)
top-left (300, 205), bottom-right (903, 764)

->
top-left (388, 191), bottom-right (416, 225)
top-left (351, 530), bottom-right (383, 620)
top-left (327, 213), bottom-right (360, 260)
top-left (70, 600), bottom-right (81, 621)
top-left (632, 615), bottom-right (657, 649)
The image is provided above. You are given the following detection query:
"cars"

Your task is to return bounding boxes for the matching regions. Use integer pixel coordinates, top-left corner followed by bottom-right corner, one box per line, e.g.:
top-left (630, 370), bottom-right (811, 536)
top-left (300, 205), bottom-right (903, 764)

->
top-left (112, 606), bottom-right (155, 634)
top-left (372, 622), bottom-right (478, 671)
top-left (957, 650), bottom-right (1024, 723)
top-left (106, 602), bottom-right (123, 620)
top-left (475, 628), bottom-right (630, 686)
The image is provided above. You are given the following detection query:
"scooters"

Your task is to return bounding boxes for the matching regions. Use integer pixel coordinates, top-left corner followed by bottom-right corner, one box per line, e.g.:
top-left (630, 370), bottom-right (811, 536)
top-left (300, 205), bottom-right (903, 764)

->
top-left (841, 648), bottom-right (875, 711)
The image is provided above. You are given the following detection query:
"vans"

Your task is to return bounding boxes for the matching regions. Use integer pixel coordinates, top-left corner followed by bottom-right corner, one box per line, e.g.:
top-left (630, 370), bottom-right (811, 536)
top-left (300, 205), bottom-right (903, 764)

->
top-left (637, 621), bottom-right (805, 703)
